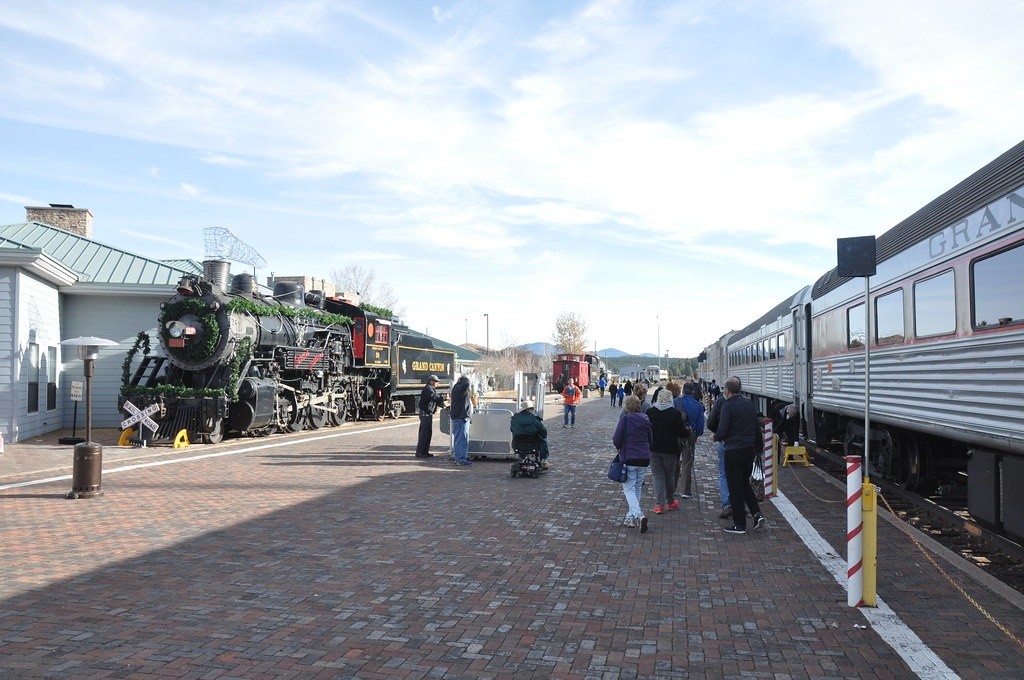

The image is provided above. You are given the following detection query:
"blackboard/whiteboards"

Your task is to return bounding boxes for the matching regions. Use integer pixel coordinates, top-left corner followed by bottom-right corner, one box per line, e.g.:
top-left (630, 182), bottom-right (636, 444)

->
top-left (70, 381), bottom-right (83, 401)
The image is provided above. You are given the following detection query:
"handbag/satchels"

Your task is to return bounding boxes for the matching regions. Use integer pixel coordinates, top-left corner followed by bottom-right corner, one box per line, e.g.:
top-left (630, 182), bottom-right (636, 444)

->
top-left (608, 454), bottom-right (627, 482)
top-left (749, 457), bottom-right (764, 501)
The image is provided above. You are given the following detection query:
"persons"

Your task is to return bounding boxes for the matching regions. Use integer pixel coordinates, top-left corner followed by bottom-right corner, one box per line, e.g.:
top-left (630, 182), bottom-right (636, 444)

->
top-left (620, 383), bottom-right (650, 420)
top-left (608, 380), bottom-right (639, 407)
top-left (674, 383), bottom-right (704, 497)
top-left (770, 402), bottom-right (800, 466)
top-left (415, 375), bottom-right (450, 458)
top-left (563, 378), bottom-right (581, 428)
top-left (612, 396), bottom-right (651, 532)
top-left (645, 389), bottom-right (687, 514)
top-left (691, 378), bottom-right (703, 402)
top-left (706, 376), bottom-right (741, 518)
top-left (643, 378), bottom-right (650, 389)
top-left (708, 379), bottom-right (720, 399)
top-left (451, 375), bottom-right (473, 466)
top-left (651, 383), bottom-right (681, 404)
top-left (710, 375), bottom-right (765, 533)
top-left (599, 376), bottom-right (607, 397)
top-left (509, 400), bottom-right (550, 468)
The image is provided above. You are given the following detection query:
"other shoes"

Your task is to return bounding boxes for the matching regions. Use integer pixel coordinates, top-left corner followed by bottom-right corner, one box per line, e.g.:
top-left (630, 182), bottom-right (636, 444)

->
top-left (719, 508), bottom-right (732, 518)
top-left (682, 494), bottom-right (693, 498)
top-left (571, 425), bottom-right (574, 428)
top-left (426, 452), bottom-right (433, 456)
top-left (563, 425), bottom-right (567, 428)
top-left (415, 452), bottom-right (427, 457)
top-left (455, 460), bottom-right (472, 466)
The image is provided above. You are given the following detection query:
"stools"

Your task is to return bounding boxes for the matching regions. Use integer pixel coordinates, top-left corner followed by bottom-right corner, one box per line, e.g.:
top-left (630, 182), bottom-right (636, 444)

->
top-left (783, 445), bottom-right (810, 467)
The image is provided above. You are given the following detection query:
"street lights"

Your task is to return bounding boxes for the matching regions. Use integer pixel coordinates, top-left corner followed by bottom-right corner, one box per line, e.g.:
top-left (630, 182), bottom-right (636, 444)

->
top-left (656, 315), bottom-right (661, 370)
top-left (483, 313), bottom-right (489, 359)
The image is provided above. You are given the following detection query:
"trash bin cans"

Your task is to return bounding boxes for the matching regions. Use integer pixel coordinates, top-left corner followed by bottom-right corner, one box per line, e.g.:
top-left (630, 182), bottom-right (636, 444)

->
top-left (583, 385), bottom-right (590, 398)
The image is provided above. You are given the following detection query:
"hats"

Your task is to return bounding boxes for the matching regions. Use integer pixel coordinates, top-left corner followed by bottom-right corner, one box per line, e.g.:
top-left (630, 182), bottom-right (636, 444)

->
top-left (519, 401), bottom-right (534, 412)
top-left (429, 375), bottom-right (441, 382)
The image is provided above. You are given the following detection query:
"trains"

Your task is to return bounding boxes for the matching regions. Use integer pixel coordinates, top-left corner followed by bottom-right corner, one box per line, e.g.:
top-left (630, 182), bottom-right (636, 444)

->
top-left (117, 258), bottom-right (456, 446)
top-left (551, 351), bottom-right (608, 394)
top-left (696, 138), bottom-right (1024, 542)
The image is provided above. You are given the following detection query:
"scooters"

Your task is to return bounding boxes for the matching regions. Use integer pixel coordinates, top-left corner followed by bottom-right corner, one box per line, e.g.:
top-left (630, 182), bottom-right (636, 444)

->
top-left (510, 432), bottom-right (547, 479)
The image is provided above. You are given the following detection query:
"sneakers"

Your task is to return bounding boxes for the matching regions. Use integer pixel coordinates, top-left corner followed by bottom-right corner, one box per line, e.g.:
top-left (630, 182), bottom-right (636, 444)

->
top-left (624, 518), bottom-right (636, 527)
top-left (753, 514), bottom-right (764, 529)
top-left (667, 500), bottom-right (679, 510)
top-left (639, 516), bottom-right (648, 533)
top-left (653, 506), bottom-right (663, 513)
top-left (724, 525), bottom-right (746, 533)
